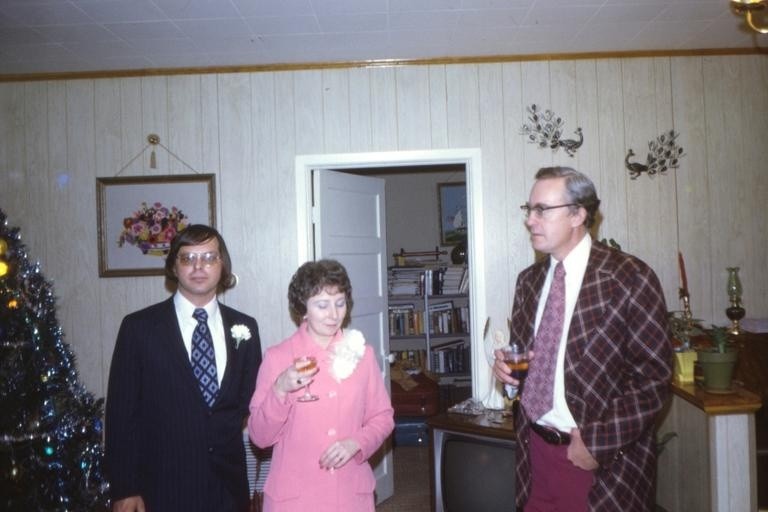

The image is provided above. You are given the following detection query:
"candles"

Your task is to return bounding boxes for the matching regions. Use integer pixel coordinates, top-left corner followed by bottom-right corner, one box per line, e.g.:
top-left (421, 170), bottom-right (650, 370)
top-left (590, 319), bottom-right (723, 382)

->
top-left (677, 252), bottom-right (691, 294)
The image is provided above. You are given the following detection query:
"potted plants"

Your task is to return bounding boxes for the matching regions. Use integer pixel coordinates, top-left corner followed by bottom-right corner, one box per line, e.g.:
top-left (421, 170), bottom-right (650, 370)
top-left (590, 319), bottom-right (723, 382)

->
top-left (699, 326), bottom-right (737, 390)
top-left (668, 313), bottom-right (699, 383)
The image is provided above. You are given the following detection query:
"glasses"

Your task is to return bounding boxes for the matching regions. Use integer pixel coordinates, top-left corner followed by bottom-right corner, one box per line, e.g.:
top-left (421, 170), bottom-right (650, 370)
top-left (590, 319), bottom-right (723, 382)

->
top-left (519, 202), bottom-right (577, 218)
top-left (176, 251), bottom-right (219, 265)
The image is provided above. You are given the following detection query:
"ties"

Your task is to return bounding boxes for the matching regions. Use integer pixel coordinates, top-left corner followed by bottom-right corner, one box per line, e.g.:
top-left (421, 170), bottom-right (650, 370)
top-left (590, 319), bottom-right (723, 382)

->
top-left (518, 261), bottom-right (566, 424)
top-left (191, 308), bottom-right (220, 407)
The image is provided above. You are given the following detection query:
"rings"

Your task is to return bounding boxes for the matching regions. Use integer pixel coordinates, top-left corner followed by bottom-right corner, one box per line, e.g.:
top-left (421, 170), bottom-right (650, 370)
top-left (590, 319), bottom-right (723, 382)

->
top-left (297, 378), bottom-right (302, 386)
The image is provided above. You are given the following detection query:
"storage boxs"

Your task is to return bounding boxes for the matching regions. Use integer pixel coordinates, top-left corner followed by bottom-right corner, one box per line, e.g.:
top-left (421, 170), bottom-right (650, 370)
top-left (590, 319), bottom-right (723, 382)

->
top-left (392, 417), bottom-right (433, 447)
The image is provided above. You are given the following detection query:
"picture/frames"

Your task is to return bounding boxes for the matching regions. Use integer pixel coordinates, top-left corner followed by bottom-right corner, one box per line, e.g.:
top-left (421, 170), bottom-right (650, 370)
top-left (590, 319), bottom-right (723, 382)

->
top-left (437, 180), bottom-right (468, 246)
top-left (95, 172), bottom-right (218, 277)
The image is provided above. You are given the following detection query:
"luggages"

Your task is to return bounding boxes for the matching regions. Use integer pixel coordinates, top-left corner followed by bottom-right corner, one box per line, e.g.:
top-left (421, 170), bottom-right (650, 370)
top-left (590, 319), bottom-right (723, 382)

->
top-left (394, 419), bottom-right (429, 447)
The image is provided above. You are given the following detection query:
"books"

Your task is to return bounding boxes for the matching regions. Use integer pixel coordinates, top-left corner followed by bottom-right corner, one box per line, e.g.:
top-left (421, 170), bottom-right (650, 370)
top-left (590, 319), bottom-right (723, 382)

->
top-left (391, 264), bottom-right (426, 296)
top-left (387, 304), bottom-right (426, 336)
top-left (429, 301), bottom-right (471, 334)
top-left (428, 264), bottom-right (469, 296)
top-left (431, 339), bottom-right (464, 351)
top-left (390, 348), bottom-right (427, 371)
top-left (431, 347), bottom-right (471, 374)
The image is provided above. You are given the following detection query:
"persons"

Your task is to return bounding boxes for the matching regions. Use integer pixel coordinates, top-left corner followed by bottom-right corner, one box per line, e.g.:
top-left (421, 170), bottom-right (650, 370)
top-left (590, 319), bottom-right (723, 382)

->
top-left (492, 163), bottom-right (675, 511)
top-left (101, 224), bottom-right (262, 512)
top-left (246, 258), bottom-right (396, 511)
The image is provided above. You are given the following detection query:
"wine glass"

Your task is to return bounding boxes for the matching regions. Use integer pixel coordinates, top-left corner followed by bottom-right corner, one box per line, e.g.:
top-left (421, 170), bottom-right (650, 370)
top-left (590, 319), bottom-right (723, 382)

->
top-left (295, 357), bottom-right (319, 401)
top-left (506, 345), bottom-right (529, 401)
